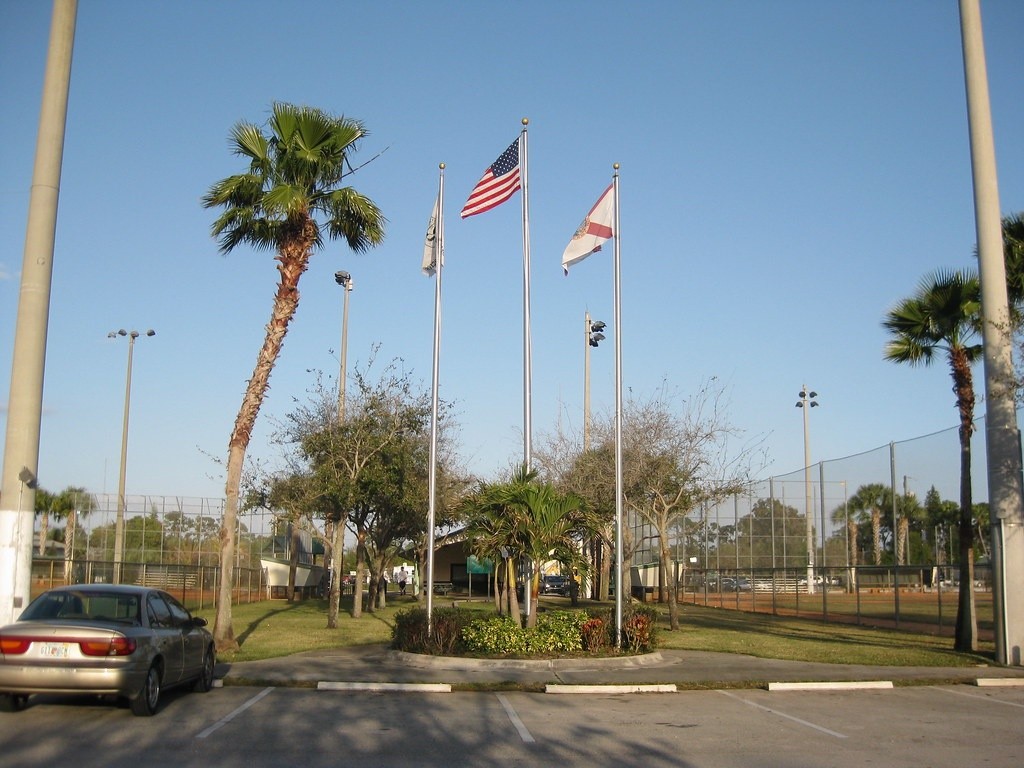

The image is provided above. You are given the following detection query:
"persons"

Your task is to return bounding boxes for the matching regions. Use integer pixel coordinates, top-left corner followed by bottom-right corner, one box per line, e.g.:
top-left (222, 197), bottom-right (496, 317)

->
top-left (342, 575), bottom-right (355, 592)
top-left (398, 566), bottom-right (408, 596)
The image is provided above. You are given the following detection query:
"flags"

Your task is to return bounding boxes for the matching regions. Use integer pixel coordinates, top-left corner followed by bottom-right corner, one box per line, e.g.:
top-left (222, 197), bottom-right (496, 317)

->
top-left (459, 135), bottom-right (522, 221)
top-left (421, 192), bottom-right (446, 279)
top-left (561, 181), bottom-right (616, 277)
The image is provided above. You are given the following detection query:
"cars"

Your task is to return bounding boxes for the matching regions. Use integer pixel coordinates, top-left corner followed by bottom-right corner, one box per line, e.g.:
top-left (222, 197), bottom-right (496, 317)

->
top-left (686, 576), bottom-right (768, 593)
top-left (796, 574), bottom-right (844, 587)
top-left (538, 574), bottom-right (579, 597)
top-left (0, 583), bottom-right (218, 718)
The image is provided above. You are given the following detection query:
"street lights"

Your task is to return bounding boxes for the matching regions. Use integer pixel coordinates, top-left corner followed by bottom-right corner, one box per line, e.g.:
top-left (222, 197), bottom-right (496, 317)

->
top-left (796, 384), bottom-right (818, 596)
top-left (334, 269), bottom-right (355, 435)
top-left (584, 311), bottom-right (622, 467)
top-left (106, 328), bottom-right (157, 586)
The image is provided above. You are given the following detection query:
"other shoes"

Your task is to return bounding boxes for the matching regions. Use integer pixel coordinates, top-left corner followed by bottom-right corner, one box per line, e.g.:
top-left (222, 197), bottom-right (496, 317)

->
top-left (401, 593), bottom-right (402, 595)
top-left (404, 590), bottom-right (405, 595)
